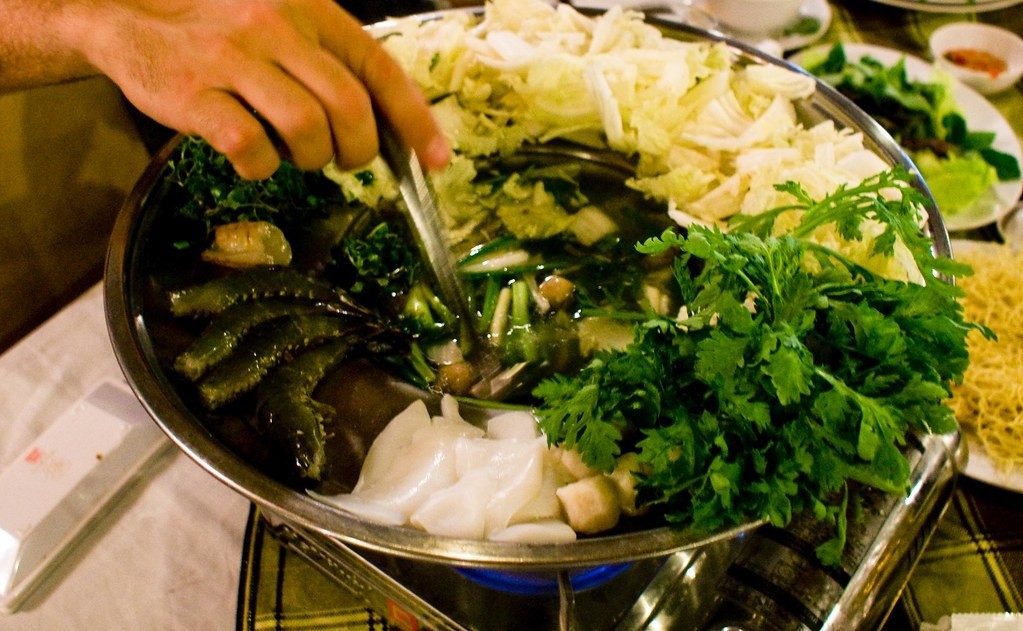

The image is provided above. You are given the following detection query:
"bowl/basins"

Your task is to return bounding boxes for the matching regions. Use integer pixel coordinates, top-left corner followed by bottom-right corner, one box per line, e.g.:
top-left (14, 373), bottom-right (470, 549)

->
top-left (929, 21), bottom-right (1023, 96)
top-left (105, 6), bottom-right (959, 565)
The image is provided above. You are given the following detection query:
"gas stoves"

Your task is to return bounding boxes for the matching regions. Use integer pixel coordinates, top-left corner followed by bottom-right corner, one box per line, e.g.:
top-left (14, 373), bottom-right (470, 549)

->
top-left (239, 393), bottom-right (1022, 630)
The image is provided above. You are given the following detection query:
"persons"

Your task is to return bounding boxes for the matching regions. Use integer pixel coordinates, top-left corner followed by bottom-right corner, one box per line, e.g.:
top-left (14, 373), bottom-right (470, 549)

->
top-left (0, 0), bottom-right (451, 356)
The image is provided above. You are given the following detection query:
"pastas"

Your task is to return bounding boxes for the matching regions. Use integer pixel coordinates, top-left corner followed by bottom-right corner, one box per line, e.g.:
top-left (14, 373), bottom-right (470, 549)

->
top-left (940, 220), bottom-right (1022, 477)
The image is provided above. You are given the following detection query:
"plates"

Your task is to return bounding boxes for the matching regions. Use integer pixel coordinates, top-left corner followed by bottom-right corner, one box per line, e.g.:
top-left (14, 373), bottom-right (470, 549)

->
top-left (780, 43), bottom-right (1023, 234)
top-left (932, 237), bottom-right (1022, 496)
top-left (872, 0), bottom-right (1023, 14)
top-left (675, 1), bottom-right (832, 55)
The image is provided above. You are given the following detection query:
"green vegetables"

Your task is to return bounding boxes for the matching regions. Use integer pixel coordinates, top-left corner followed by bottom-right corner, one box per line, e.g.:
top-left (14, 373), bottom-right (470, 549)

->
top-left (806, 41), bottom-right (1021, 218)
